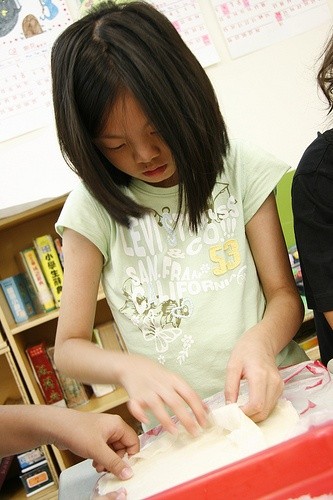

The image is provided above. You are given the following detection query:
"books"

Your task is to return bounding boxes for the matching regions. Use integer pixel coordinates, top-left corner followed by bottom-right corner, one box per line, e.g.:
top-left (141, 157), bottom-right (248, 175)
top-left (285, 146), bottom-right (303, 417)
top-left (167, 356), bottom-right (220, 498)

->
top-left (0, 232), bottom-right (129, 404)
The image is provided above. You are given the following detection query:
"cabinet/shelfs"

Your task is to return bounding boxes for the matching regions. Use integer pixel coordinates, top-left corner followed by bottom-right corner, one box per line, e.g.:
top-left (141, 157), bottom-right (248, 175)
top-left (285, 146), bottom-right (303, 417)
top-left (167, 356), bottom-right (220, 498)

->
top-left (1, 192), bottom-right (142, 500)
top-left (1, 334), bottom-right (59, 500)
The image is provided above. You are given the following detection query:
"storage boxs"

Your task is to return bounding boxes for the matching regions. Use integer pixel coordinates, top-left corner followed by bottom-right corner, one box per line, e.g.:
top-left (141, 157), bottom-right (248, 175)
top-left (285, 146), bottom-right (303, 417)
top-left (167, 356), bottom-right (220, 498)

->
top-left (20, 465), bottom-right (55, 497)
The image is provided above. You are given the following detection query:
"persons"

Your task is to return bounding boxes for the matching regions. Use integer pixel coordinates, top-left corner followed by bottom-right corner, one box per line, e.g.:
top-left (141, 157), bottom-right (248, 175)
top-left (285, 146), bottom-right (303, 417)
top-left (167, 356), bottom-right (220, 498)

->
top-left (1, 405), bottom-right (141, 500)
top-left (53, 0), bottom-right (309, 425)
top-left (291, 30), bottom-right (333, 371)
